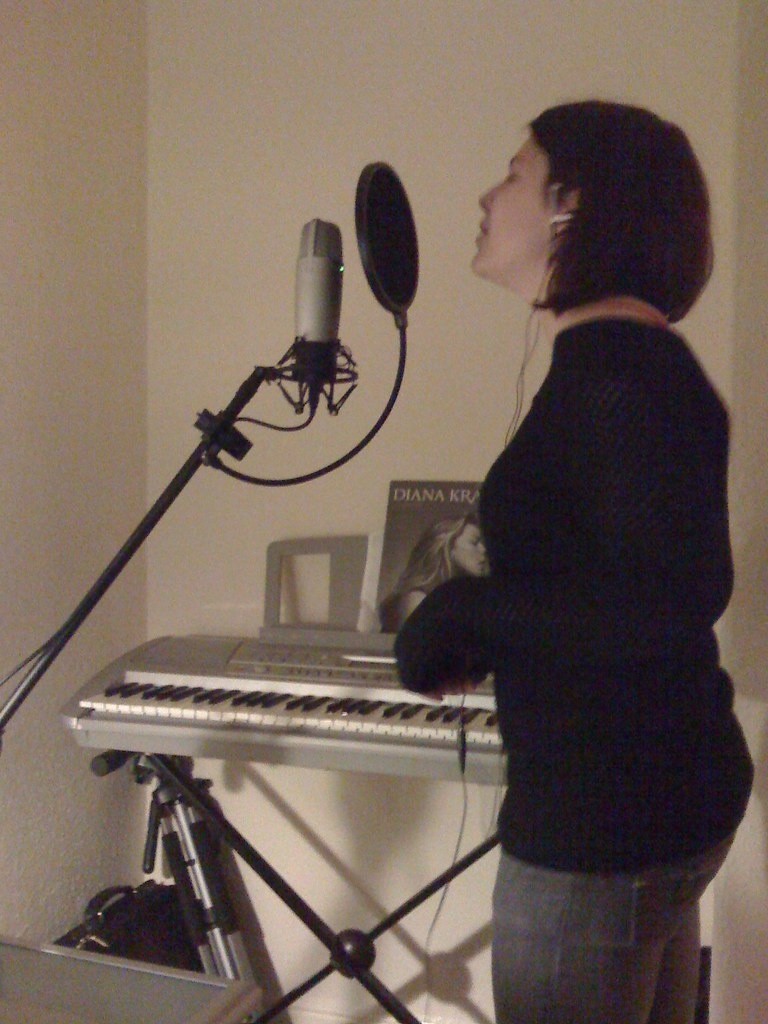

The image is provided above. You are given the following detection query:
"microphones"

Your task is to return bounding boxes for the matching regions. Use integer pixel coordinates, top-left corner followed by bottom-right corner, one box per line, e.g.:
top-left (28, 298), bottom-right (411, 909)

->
top-left (293, 216), bottom-right (342, 412)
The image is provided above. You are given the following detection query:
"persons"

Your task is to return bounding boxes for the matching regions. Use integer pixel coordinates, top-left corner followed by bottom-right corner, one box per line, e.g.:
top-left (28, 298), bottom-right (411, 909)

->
top-left (380, 513), bottom-right (486, 634)
top-left (391, 102), bottom-right (755, 1024)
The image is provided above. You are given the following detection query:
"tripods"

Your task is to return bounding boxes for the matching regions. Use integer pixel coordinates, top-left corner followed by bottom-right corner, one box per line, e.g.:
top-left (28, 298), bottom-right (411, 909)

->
top-left (84, 749), bottom-right (253, 982)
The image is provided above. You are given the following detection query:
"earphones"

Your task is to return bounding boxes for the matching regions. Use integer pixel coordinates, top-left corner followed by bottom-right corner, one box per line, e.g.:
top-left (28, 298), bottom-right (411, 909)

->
top-left (551, 209), bottom-right (577, 226)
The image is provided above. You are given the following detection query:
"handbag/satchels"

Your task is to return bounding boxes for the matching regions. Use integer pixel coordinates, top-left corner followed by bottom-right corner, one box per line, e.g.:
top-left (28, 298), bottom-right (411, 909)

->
top-left (72, 880), bottom-right (205, 975)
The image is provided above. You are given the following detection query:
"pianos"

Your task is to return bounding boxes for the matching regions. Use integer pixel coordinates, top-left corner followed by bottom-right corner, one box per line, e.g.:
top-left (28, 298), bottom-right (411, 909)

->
top-left (58, 632), bottom-right (514, 1024)
